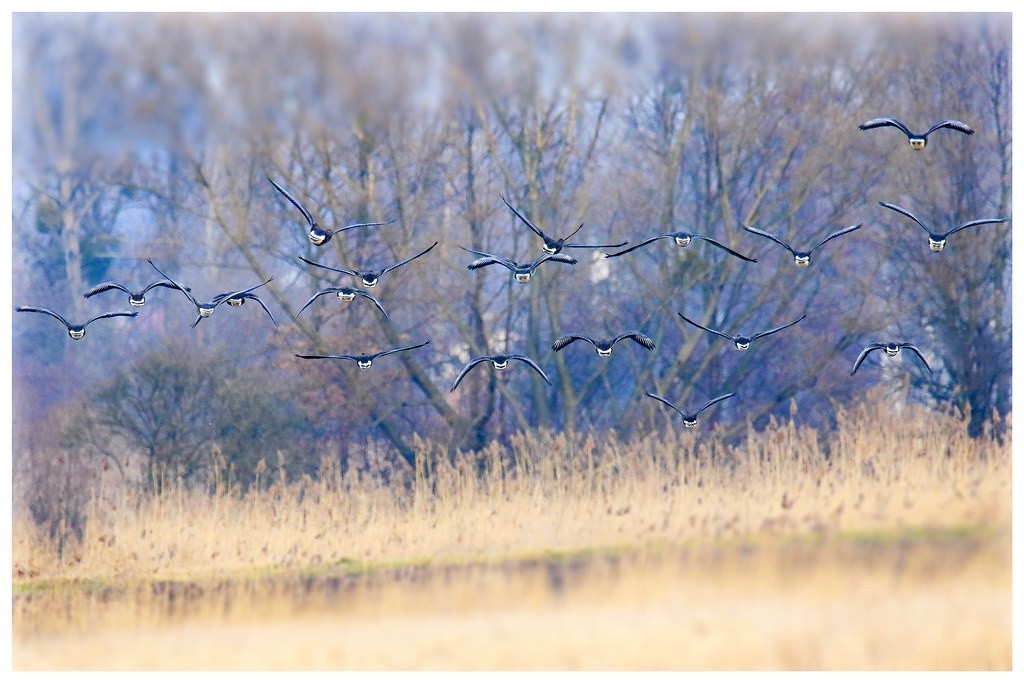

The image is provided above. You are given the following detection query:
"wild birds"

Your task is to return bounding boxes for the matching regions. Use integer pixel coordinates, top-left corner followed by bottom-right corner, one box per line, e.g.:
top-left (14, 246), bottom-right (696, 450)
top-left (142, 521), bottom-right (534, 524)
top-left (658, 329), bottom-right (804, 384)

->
top-left (449, 353), bottom-right (554, 392)
top-left (550, 329), bottom-right (657, 359)
top-left (14, 257), bottom-right (279, 340)
top-left (293, 339), bottom-right (434, 369)
top-left (848, 340), bottom-right (935, 379)
top-left (876, 199), bottom-right (1009, 254)
top-left (643, 389), bottom-right (739, 429)
top-left (858, 114), bottom-right (976, 151)
top-left (264, 175), bottom-right (439, 323)
top-left (677, 311), bottom-right (808, 352)
top-left (452, 192), bottom-right (864, 285)
